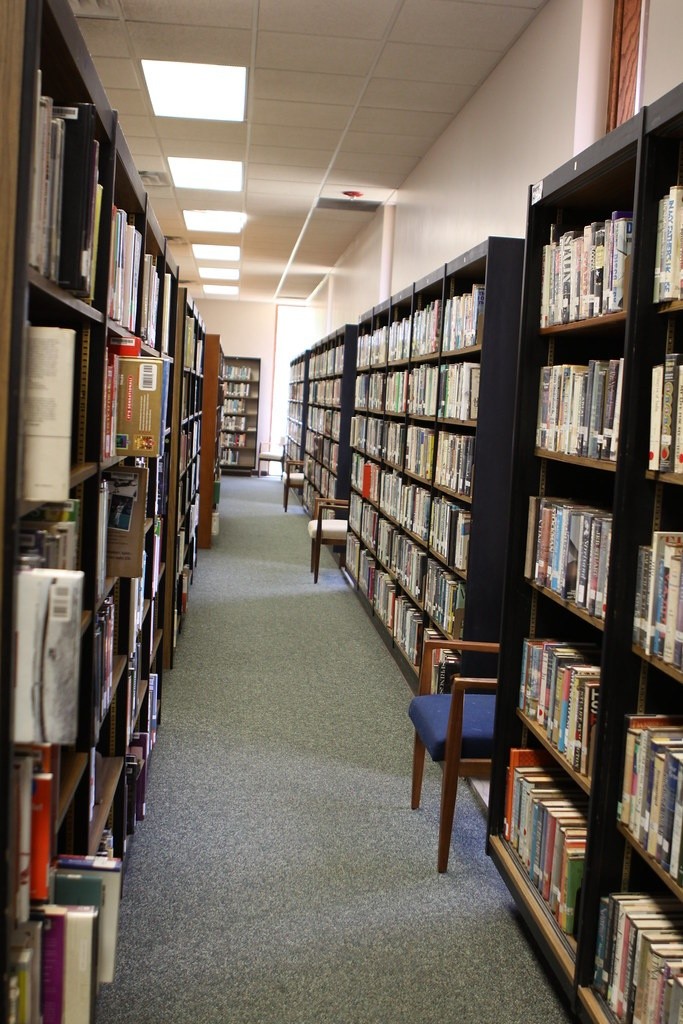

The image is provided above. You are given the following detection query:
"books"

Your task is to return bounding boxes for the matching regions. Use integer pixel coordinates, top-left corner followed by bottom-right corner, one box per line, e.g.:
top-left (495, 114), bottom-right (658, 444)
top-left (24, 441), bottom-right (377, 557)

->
top-left (2, 63), bottom-right (221, 1024)
top-left (284, 184), bottom-right (681, 1024)
top-left (221, 358), bottom-right (252, 465)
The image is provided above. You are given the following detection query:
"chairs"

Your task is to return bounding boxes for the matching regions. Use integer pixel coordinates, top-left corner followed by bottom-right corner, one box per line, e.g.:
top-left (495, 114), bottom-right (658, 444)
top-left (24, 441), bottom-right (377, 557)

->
top-left (258, 441), bottom-right (285, 481)
top-left (408, 641), bottom-right (500, 873)
top-left (308, 498), bottom-right (349, 585)
top-left (282, 461), bottom-right (306, 512)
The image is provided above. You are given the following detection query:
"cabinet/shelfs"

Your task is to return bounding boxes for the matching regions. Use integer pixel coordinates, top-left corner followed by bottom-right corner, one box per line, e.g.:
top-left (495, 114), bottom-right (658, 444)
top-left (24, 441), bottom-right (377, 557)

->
top-left (285, 82), bottom-right (683, 1024)
top-left (0, 0), bottom-right (260, 1024)
